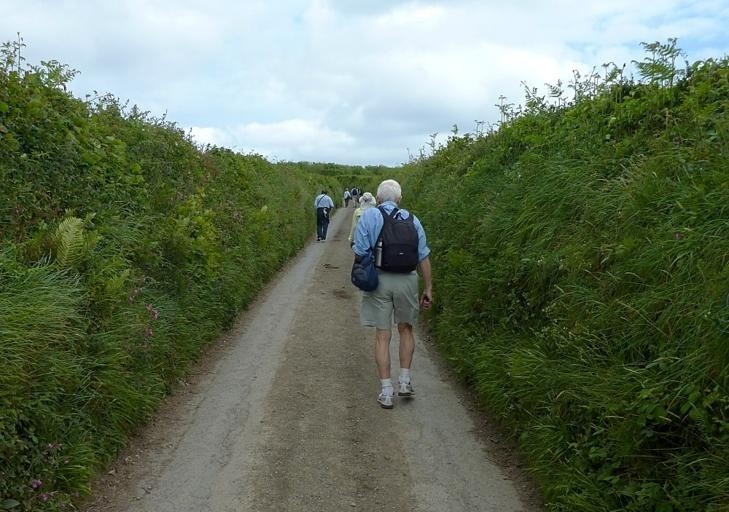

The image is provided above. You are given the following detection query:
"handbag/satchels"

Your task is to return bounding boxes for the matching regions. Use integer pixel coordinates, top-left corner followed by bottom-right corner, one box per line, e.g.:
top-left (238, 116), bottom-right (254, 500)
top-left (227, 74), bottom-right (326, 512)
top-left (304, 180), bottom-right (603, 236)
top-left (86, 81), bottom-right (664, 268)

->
top-left (351, 250), bottom-right (378, 290)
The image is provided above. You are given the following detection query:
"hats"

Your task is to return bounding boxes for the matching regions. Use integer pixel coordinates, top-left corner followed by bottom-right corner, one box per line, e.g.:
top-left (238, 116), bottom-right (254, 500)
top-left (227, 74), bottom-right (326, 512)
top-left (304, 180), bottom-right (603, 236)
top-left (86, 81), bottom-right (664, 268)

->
top-left (359, 192), bottom-right (376, 205)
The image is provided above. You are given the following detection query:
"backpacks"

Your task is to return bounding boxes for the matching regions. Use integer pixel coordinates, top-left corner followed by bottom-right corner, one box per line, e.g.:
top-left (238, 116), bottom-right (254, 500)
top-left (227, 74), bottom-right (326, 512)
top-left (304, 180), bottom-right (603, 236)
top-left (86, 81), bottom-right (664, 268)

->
top-left (377, 206), bottom-right (418, 273)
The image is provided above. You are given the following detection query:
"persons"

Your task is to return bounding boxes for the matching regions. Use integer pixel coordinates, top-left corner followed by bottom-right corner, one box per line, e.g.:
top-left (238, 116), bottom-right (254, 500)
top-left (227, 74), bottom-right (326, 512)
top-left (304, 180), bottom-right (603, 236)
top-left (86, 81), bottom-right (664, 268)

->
top-left (344, 184), bottom-right (362, 209)
top-left (314, 190), bottom-right (334, 242)
top-left (352, 179), bottom-right (434, 412)
top-left (348, 192), bottom-right (376, 250)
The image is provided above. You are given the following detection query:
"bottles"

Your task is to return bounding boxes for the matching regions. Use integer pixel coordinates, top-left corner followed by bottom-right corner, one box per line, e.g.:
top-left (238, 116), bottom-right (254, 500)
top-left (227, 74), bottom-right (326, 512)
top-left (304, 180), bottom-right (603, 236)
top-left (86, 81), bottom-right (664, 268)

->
top-left (376, 238), bottom-right (382, 267)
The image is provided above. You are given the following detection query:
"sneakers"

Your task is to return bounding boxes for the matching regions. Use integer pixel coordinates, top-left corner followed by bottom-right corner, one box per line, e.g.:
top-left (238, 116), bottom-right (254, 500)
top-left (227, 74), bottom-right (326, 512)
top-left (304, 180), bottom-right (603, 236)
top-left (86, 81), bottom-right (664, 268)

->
top-left (377, 393), bottom-right (393, 408)
top-left (398, 382), bottom-right (415, 396)
